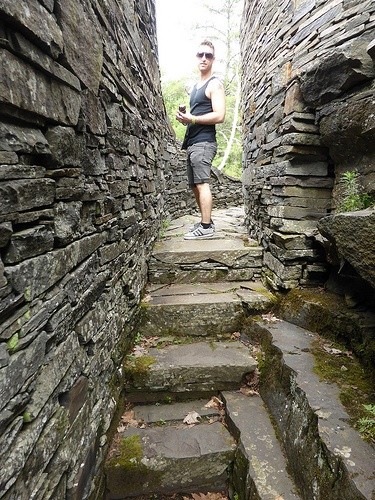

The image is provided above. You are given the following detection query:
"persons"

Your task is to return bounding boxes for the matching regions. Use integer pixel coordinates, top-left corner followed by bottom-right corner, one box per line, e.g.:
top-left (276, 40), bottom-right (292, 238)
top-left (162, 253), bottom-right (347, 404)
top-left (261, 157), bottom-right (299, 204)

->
top-left (176, 40), bottom-right (226, 240)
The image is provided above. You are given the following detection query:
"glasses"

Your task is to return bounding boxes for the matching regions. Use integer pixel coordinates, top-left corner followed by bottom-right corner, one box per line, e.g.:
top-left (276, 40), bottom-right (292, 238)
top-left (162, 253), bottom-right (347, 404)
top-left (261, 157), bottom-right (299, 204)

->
top-left (196, 52), bottom-right (213, 60)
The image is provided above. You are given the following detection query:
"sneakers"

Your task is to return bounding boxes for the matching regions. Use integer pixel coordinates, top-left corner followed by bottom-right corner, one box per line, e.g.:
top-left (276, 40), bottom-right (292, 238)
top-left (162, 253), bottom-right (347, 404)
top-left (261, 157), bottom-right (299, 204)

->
top-left (183, 224), bottom-right (215, 239)
top-left (189, 218), bottom-right (215, 233)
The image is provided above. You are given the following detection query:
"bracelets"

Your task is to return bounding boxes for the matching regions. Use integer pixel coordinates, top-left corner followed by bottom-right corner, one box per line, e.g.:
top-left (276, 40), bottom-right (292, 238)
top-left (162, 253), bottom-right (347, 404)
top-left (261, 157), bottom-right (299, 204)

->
top-left (192, 115), bottom-right (196, 125)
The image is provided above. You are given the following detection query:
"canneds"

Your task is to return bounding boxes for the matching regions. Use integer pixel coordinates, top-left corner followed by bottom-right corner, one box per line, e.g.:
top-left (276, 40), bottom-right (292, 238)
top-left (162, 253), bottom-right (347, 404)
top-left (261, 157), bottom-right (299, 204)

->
top-left (179, 105), bottom-right (186, 117)
